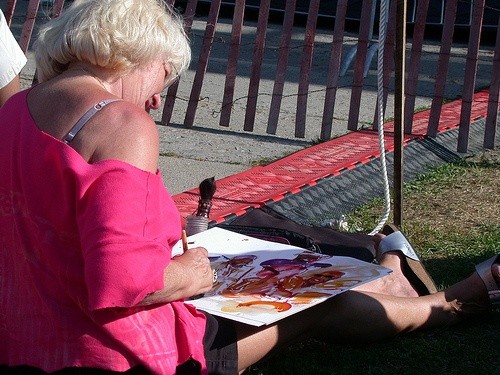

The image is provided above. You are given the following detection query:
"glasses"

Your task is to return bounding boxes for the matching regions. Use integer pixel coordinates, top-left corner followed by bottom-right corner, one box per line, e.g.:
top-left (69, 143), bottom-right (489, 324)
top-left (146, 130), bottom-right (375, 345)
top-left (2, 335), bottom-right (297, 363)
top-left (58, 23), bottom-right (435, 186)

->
top-left (161, 59), bottom-right (182, 97)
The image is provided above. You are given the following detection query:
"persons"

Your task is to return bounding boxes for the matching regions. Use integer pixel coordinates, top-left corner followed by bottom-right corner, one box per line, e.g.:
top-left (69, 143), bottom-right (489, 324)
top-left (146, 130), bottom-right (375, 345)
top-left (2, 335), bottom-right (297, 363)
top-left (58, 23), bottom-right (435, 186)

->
top-left (1, 9), bottom-right (26, 108)
top-left (0, 0), bottom-right (500, 375)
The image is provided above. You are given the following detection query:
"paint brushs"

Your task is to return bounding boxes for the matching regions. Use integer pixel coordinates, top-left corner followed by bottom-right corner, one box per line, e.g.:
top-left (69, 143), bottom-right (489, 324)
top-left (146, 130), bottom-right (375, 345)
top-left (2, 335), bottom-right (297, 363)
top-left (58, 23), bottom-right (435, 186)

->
top-left (181, 228), bottom-right (190, 254)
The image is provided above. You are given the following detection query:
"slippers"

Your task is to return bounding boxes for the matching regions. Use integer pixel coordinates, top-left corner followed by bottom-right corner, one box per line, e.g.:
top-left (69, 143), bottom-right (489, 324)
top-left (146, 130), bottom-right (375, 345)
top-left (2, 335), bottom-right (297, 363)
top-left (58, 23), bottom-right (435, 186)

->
top-left (476, 253), bottom-right (500, 310)
top-left (374, 222), bottom-right (438, 296)
